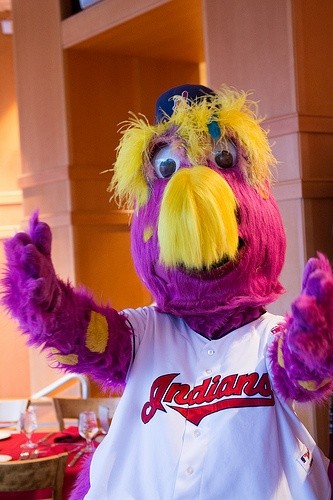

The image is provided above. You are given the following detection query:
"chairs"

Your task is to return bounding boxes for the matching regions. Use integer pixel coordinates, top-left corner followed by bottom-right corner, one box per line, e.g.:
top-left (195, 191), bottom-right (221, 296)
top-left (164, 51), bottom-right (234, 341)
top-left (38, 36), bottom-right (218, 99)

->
top-left (0, 453), bottom-right (67, 500)
top-left (53, 397), bottom-right (100, 430)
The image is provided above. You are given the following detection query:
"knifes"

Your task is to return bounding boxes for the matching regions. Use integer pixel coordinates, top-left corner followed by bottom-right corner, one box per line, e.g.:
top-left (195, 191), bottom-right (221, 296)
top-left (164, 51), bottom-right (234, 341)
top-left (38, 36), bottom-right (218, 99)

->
top-left (67, 448), bottom-right (86, 467)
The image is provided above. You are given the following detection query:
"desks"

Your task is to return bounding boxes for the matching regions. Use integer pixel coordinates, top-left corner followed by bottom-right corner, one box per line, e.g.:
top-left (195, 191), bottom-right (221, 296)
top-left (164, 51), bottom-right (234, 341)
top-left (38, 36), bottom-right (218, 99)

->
top-left (0, 432), bottom-right (106, 500)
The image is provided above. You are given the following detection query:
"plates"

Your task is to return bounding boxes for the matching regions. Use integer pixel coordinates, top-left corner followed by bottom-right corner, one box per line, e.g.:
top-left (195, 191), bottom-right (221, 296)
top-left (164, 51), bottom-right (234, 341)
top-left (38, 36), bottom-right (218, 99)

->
top-left (0, 455), bottom-right (12, 462)
top-left (0, 432), bottom-right (11, 440)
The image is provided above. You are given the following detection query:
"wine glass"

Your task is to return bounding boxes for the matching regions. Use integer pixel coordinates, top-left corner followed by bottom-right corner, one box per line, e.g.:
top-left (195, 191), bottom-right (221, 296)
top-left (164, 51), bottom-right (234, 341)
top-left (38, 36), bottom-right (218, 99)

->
top-left (78, 411), bottom-right (99, 452)
top-left (19, 410), bottom-right (38, 448)
